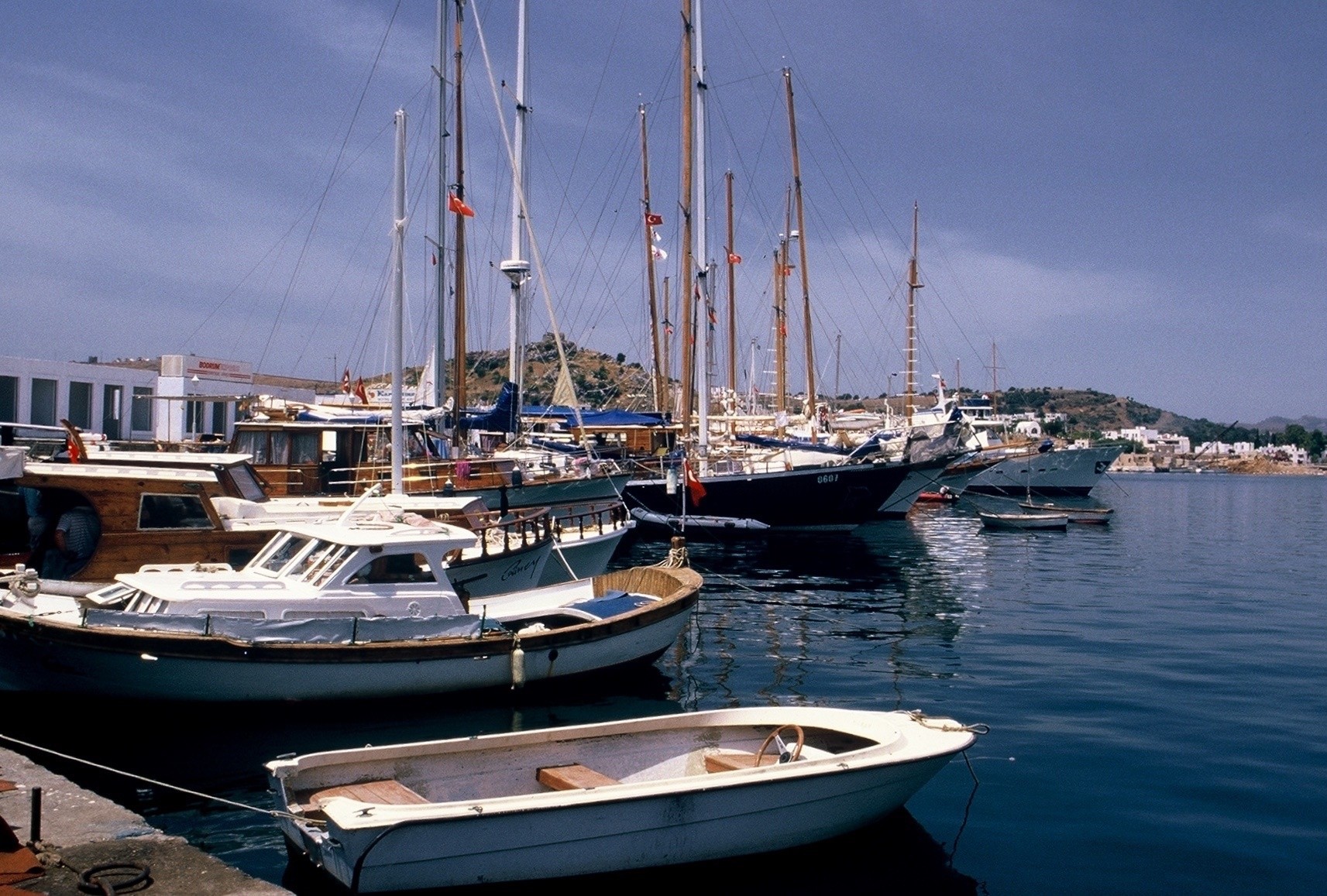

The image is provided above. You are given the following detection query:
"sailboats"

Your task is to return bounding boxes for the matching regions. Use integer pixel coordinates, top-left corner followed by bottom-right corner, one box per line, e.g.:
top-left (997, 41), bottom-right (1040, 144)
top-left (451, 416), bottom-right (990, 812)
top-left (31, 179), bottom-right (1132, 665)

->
top-left (204, 0), bottom-right (1125, 521)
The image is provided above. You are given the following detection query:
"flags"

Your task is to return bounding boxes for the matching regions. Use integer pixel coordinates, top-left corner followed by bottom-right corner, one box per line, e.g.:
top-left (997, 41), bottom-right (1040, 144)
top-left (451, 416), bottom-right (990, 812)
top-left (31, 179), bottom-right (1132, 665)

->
top-left (446, 188), bottom-right (477, 217)
top-left (776, 260), bottom-right (791, 277)
top-left (724, 246), bottom-right (742, 265)
top-left (341, 369), bottom-right (370, 406)
top-left (645, 212), bottom-right (664, 225)
top-left (683, 456), bottom-right (705, 506)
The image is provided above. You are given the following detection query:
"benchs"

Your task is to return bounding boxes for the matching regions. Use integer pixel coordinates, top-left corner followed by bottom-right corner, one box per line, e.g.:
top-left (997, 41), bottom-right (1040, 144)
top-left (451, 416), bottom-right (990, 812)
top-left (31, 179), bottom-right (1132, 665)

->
top-left (308, 779), bottom-right (432, 805)
top-left (536, 763), bottom-right (623, 792)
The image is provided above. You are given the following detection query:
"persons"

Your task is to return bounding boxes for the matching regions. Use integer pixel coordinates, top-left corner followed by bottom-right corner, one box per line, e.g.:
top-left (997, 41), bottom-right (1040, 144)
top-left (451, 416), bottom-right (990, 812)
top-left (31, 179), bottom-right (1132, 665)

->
top-left (55, 507), bottom-right (95, 568)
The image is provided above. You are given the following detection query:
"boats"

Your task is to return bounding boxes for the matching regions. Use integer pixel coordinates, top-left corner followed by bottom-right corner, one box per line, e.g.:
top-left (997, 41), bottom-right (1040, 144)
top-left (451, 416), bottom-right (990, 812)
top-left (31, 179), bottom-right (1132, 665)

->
top-left (0, 462), bottom-right (557, 599)
top-left (0, 483), bottom-right (704, 705)
top-left (63, 449), bottom-right (637, 588)
top-left (977, 512), bottom-right (1068, 530)
top-left (264, 706), bottom-right (977, 896)
